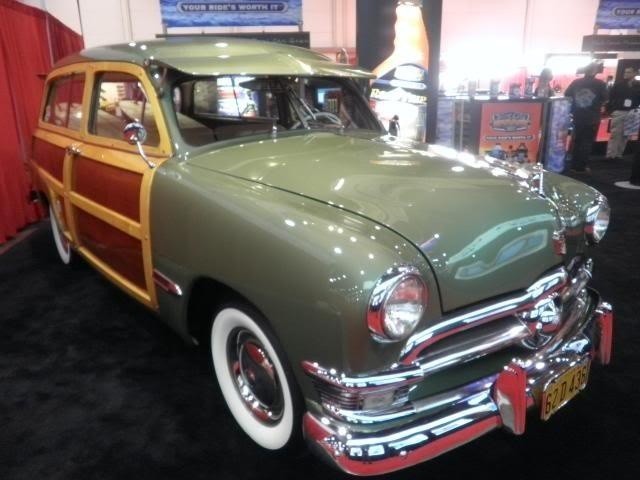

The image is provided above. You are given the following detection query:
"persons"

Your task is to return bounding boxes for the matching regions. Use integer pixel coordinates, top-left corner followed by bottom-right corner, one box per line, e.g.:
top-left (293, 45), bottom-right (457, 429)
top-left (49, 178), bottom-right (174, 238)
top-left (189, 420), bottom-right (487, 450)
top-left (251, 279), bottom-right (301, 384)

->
top-left (614, 123), bottom-right (640, 190)
top-left (535, 68), bottom-right (555, 97)
top-left (598, 65), bottom-right (640, 162)
top-left (564, 62), bottom-right (609, 172)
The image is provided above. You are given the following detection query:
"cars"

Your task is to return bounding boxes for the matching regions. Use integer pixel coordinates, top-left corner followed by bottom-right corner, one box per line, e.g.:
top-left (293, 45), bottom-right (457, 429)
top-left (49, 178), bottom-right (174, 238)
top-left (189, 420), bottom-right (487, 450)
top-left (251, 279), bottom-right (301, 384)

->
top-left (31, 36), bottom-right (616, 476)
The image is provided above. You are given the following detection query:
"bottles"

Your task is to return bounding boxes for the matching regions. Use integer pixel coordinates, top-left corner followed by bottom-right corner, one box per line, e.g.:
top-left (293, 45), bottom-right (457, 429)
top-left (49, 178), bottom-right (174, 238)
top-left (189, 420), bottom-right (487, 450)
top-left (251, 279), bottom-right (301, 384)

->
top-left (508, 81), bottom-right (519, 100)
top-left (523, 76), bottom-right (535, 98)
top-left (467, 79), bottom-right (476, 101)
top-left (489, 81), bottom-right (498, 100)
top-left (366, 0), bottom-right (429, 147)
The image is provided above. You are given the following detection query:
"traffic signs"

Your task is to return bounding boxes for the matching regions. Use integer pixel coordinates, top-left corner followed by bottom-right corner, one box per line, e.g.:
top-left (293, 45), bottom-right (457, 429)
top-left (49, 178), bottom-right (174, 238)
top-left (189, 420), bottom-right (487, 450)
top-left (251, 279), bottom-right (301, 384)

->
top-left (583, 35), bottom-right (639, 52)
